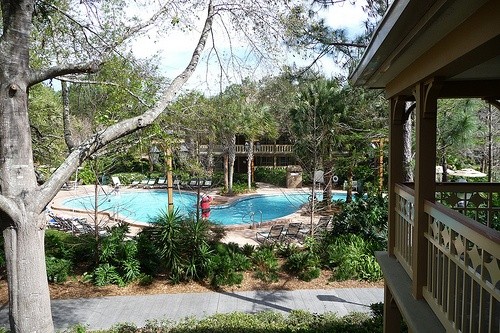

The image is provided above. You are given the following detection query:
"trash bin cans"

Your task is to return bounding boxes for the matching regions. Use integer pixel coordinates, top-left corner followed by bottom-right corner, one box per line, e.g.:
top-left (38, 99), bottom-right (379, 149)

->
top-left (285, 164), bottom-right (303, 189)
